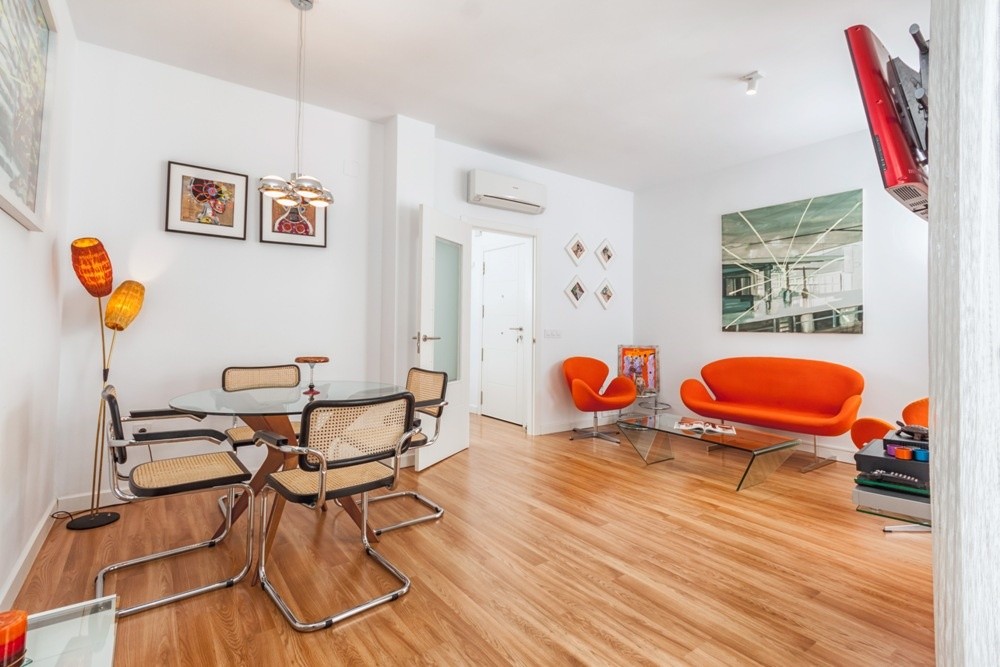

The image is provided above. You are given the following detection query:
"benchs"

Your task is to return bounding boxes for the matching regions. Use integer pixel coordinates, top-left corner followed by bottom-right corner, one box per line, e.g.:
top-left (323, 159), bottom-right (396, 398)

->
top-left (679, 356), bottom-right (864, 475)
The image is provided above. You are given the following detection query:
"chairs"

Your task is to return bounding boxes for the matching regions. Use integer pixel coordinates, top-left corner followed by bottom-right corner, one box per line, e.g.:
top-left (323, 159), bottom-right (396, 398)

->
top-left (92, 353), bottom-right (448, 634)
top-left (562, 356), bottom-right (639, 444)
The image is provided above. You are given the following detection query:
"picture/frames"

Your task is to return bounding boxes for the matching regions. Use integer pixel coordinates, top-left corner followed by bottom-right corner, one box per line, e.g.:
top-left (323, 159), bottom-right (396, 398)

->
top-left (261, 188), bottom-right (329, 248)
top-left (1, 1), bottom-right (57, 230)
top-left (564, 235), bottom-right (618, 308)
top-left (166, 161), bottom-right (249, 240)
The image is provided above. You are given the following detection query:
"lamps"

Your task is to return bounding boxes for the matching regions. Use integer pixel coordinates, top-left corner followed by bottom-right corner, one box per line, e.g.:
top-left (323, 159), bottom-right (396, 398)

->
top-left (262, 0), bottom-right (330, 208)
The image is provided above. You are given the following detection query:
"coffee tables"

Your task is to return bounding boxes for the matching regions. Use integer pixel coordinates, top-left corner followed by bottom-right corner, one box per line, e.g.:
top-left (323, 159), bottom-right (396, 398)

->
top-left (616, 413), bottom-right (801, 492)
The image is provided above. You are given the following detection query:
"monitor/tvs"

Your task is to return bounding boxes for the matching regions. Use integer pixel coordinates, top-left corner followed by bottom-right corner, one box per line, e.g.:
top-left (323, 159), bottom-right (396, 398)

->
top-left (842, 25), bottom-right (932, 218)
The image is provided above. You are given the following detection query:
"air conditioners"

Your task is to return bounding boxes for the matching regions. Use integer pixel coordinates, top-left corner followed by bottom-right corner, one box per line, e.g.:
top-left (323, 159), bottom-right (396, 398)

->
top-left (469, 168), bottom-right (546, 215)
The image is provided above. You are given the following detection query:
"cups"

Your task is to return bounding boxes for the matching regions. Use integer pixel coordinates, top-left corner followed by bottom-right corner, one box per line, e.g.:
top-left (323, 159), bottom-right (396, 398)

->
top-left (888, 444), bottom-right (900, 455)
top-left (0, 610), bottom-right (28, 667)
top-left (895, 446), bottom-right (912, 460)
top-left (913, 449), bottom-right (929, 462)
